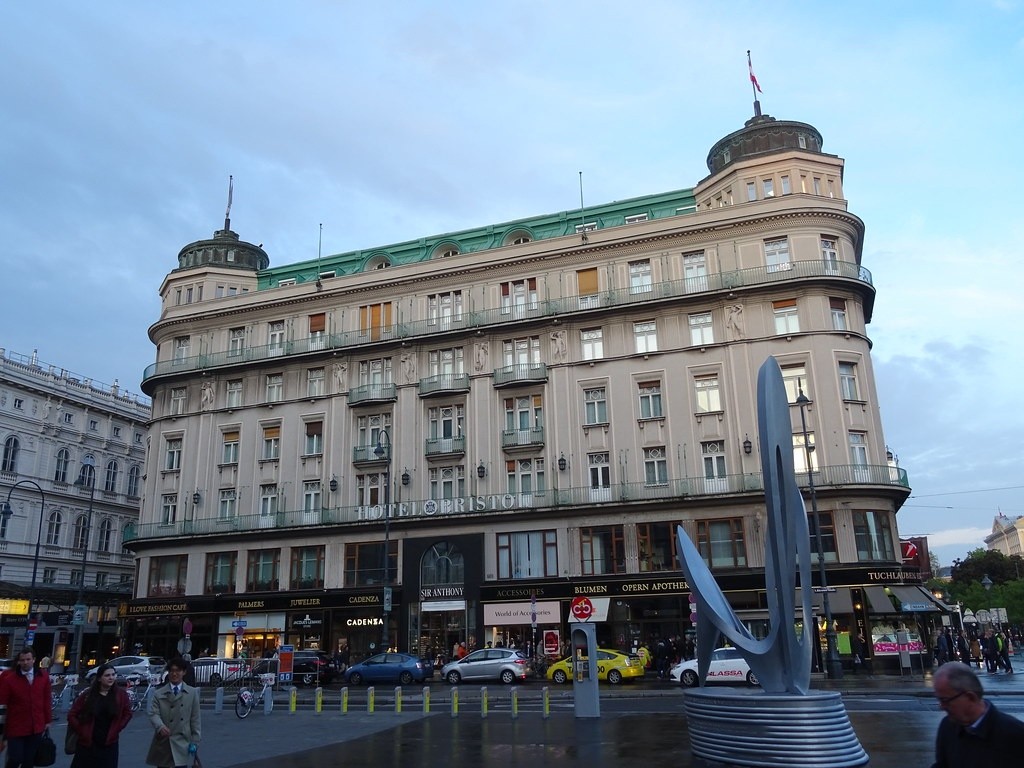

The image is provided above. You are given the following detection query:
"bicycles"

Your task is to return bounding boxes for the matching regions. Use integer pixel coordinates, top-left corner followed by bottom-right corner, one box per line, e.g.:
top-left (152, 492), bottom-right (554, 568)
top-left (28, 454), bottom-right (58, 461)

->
top-left (125, 673), bottom-right (162, 712)
top-left (50, 673), bottom-right (80, 711)
top-left (234, 670), bottom-right (275, 719)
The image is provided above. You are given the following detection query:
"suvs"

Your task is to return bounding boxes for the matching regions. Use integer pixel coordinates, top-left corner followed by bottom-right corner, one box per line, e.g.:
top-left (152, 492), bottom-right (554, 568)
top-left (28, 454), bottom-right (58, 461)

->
top-left (254, 649), bottom-right (338, 685)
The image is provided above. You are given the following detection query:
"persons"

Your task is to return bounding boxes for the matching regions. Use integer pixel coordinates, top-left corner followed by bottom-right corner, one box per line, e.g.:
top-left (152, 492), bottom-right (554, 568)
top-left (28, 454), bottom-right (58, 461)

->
top-left (0, 648), bottom-right (53, 768)
top-left (550, 331), bottom-right (565, 362)
top-left (264, 646), bottom-right (279, 658)
top-left (400, 353), bottom-right (415, 383)
top-left (201, 381), bottom-right (213, 410)
top-left (386, 647), bottom-right (398, 653)
top-left (430, 645), bottom-right (436, 670)
top-left (727, 304), bottom-right (744, 340)
top-left (476, 343), bottom-right (488, 372)
top-left (457, 641), bottom-right (466, 660)
top-left (57, 399), bottom-right (65, 425)
top-left (340, 646), bottom-right (349, 678)
top-left (81, 653), bottom-right (91, 667)
top-left (601, 634), bottom-right (730, 678)
top-left (453, 643), bottom-right (460, 661)
top-left (929, 661), bottom-right (1024, 768)
top-left (485, 638), bottom-right (572, 670)
top-left (935, 627), bottom-right (1021, 673)
top-left (67, 664), bottom-right (133, 768)
top-left (34, 612), bottom-right (47, 627)
top-left (334, 362), bottom-right (347, 392)
top-left (43, 396), bottom-right (52, 419)
top-left (145, 646), bottom-right (211, 768)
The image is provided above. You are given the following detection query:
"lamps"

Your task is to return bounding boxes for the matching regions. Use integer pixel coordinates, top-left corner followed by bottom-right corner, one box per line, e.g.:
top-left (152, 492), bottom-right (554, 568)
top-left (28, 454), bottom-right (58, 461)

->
top-left (557, 453), bottom-right (566, 472)
top-left (477, 459), bottom-right (485, 480)
top-left (743, 433), bottom-right (752, 456)
top-left (330, 473), bottom-right (338, 493)
top-left (402, 466), bottom-right (409, 487)
top-left (192, 487), bottom-right (201, 504)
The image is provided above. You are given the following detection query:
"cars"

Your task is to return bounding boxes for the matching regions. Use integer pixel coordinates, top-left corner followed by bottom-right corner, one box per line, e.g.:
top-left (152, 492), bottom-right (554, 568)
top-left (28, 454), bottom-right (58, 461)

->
top-left (344, 652), bottom-right (435, 684)
top-left (668, 646), bottom-right (761, 687)
top-left (160, 656), bottom-right (253, 687)
top-left (546, 648), bottom-right (645, 684)
top-left (954, 639), bottom-right (984, 661)
top-left (0, 659), bottom-right (16, 676)
top-left (440, 648), bottom-right (535, 685)
top-left (85, 655), bottom-right (166, 686)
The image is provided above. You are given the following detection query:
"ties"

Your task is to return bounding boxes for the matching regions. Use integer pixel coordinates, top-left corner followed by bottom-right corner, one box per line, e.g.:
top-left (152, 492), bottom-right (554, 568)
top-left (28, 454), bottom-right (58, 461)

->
top-left (174, 686), bottom-right (179, 697)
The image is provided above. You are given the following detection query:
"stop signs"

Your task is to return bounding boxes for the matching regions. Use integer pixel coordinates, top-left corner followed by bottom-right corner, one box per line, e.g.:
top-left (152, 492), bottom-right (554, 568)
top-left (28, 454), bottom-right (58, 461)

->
top-left (29, 619), bottom-right (38, 630)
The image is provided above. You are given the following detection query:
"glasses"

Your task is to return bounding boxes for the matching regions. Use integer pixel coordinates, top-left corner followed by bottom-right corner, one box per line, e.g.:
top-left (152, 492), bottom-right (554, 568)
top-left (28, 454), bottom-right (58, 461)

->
top-left (937, 689), bottom-right (974, 708)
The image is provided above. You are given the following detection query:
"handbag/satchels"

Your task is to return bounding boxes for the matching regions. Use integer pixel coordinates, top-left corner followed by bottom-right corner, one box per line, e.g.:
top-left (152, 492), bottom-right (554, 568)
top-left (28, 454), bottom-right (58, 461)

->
top-left (29, 728), bottom-right (56, 766)
top-left (65, 723), bottom-right (78, 755)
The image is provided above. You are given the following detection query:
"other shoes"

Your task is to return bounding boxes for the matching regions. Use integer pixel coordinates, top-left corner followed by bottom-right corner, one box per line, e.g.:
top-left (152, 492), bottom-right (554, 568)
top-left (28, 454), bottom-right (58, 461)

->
top-left (987, 669), bottom-right (1013, 675)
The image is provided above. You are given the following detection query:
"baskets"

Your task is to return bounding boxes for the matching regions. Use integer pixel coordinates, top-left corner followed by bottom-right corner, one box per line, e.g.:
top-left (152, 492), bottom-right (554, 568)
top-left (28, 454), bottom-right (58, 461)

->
top-left (262, 673), bottom-right (275, 685)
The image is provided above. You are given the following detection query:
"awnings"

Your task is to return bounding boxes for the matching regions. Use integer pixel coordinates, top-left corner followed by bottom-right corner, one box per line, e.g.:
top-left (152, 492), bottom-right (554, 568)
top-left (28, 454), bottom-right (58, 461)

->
top-left (794, 585), bottom-right (953, 616)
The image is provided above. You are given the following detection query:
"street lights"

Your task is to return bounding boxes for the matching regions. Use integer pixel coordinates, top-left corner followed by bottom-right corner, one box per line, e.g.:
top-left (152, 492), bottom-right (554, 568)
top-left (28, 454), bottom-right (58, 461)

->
top-left (1, 480), bottom-right (45, 648)
top-left (981, 573), bottom-right (993, 609)
top-left (74, 464), bottom-right (96, 612)
top-left (796, 375), bottom-right (845, 680)
top-left (373, 428), bottom-right (392, 652)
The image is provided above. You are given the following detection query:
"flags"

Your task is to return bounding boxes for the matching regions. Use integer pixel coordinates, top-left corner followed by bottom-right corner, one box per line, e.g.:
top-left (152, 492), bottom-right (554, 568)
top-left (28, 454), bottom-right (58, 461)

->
top-left (749, 59), bottom-right (763, 93)
top-left (903, 541), bottom-right (918, 558)
